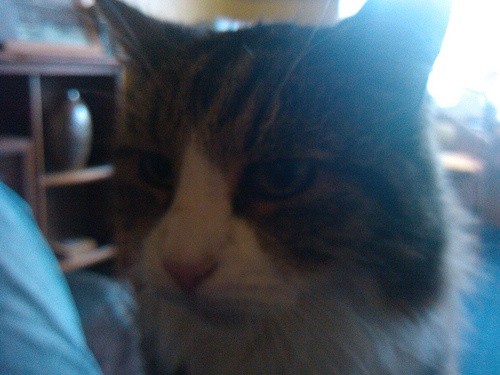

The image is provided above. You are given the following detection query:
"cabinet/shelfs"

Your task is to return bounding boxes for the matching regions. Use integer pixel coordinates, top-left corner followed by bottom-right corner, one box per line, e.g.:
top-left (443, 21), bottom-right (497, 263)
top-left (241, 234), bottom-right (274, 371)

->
top-left (0, 41), bottom-right (124, 277)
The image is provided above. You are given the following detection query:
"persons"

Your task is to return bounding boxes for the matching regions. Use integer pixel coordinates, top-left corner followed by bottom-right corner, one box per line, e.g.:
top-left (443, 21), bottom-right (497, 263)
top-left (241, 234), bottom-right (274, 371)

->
top-left (0, 181), bottom-right (104, 375)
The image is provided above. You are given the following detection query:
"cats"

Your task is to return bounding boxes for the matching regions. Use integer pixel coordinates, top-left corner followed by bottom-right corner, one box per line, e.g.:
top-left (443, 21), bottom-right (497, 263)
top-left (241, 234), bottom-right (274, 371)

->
top-left (90, 0), bottom-right (475, 375)
